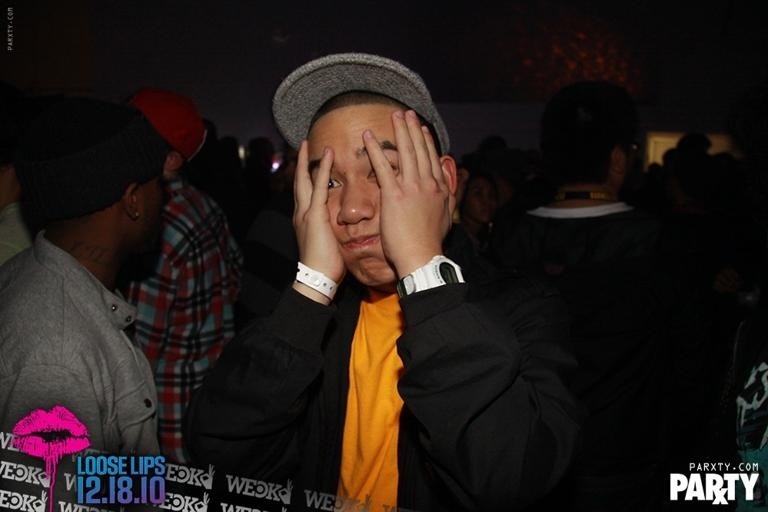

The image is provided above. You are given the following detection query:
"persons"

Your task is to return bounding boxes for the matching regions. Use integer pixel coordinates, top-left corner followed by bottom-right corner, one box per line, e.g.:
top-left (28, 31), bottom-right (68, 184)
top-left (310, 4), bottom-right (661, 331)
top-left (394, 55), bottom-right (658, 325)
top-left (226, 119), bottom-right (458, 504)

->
top-left (0, 50), bottom-right (768, 512)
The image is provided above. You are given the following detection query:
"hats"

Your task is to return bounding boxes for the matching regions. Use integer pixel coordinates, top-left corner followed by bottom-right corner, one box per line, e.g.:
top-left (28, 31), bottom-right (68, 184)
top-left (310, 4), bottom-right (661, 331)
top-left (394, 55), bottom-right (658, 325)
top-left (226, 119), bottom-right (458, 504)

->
top-left (124, 86), bottom-right (208, 164)
top-left (14, 97), bottom-right (169, 222)
top-left (272, 52), bottom-right (452, 162)
